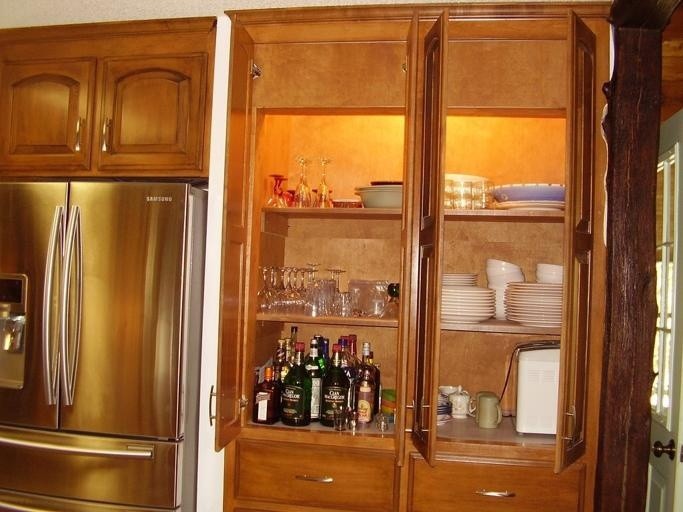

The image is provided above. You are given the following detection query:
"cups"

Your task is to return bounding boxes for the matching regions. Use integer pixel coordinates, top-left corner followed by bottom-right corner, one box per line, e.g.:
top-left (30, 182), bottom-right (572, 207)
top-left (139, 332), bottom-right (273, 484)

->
top-left (334, 409), bottom-right (349, 431)
top-left (305, 279), bottom-right (394, 317)
top-left (376, 416), bottom-right (388, 432)
top-left (445, 179), bottom-right (495, 211)
top-left (449, 385), bottom-right (502, 429)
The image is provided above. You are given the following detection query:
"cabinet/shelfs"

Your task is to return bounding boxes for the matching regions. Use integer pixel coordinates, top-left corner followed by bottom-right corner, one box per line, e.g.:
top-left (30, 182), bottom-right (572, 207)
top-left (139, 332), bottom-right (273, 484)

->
top-left (209, 0), bottom-right (614, 512)
top-left (0, 16), bottom-right (218, 186)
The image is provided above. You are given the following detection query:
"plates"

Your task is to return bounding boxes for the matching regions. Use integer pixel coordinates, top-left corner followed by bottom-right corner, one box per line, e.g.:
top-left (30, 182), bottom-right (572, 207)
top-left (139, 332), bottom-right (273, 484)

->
top-left (440, 273), bottom-right (562, 328)
top-left (491, 184), bottom-right (569, 212)
top-left (437, 385), bottom-right (453, 427)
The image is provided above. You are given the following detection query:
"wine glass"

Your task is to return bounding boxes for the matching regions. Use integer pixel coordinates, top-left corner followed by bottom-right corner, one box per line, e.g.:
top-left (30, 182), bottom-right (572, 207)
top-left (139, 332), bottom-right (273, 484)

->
top-left (257, 262), bottom-right (318, 315)
top-left (325, 268), bottom-right (346, 294)
top-left (266, 157), bottom-right (336, 209)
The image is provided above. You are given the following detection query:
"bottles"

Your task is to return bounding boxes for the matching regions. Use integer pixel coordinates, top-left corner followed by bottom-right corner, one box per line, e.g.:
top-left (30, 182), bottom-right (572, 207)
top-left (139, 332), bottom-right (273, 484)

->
top-left (254, 326), bottom-right (379, 424)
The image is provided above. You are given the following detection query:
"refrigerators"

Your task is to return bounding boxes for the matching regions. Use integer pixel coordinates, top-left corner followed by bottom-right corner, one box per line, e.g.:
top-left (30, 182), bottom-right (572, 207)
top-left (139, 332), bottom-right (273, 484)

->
top-left (0, 181), bottom-right (208, 512)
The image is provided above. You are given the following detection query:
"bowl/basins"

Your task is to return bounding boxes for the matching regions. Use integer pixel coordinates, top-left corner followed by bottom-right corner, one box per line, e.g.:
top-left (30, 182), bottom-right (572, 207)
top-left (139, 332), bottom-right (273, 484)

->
top-left (355, 184), bottom-right (407, 210)
top-left (486, 258), bottom-right (563, 273)
top-left (380, 388), bottom-right (397, 414)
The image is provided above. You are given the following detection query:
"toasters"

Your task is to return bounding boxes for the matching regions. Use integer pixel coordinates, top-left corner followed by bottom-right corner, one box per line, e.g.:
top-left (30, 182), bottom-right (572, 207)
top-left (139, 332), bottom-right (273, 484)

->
top-left (513, 338), bottom-right (560, 435)
